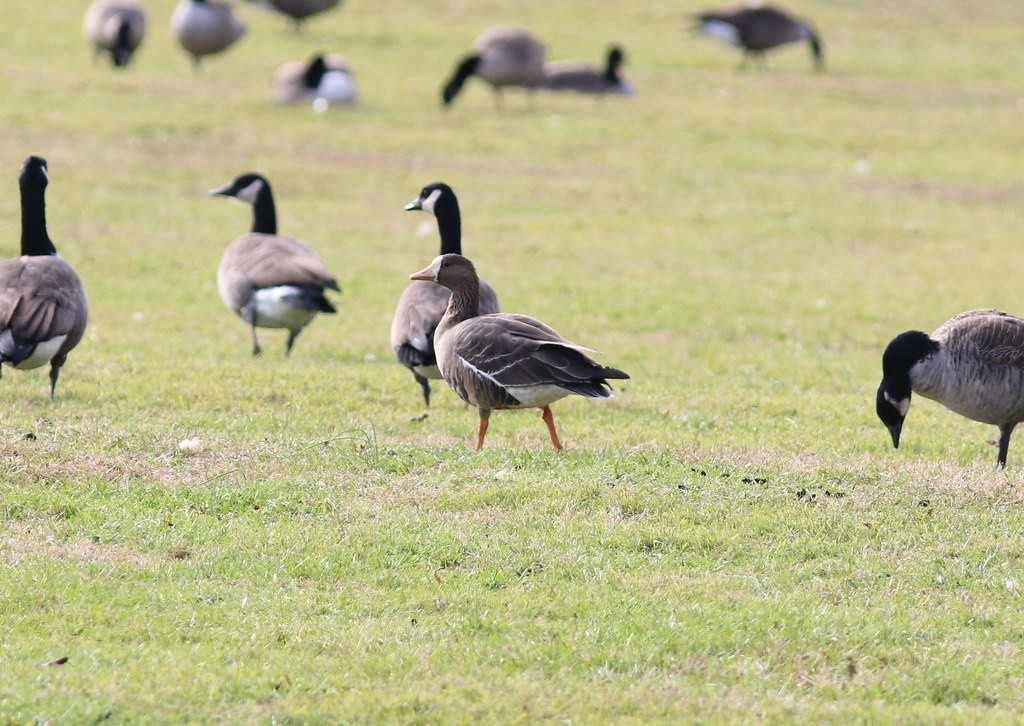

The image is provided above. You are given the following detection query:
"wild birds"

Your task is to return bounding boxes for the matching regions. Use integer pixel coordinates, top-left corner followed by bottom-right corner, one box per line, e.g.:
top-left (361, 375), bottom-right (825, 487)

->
top-left (388, 179), bottom-right (631, 455)
top-left (876, 306), bottom-right (1024, 471)
top-left (207, 172), bottom-right (344, 361)
top-left (0, 155), bottom-right (90, 402)
top-left (82, 0), bottom-right (829, 117)
